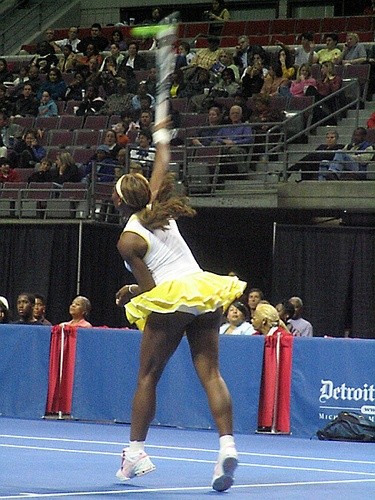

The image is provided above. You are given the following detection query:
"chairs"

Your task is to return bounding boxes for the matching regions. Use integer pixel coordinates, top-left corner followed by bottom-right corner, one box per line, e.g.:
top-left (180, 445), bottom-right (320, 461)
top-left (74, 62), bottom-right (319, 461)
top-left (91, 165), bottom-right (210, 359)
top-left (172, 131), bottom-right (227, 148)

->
top-left (189, 21), bottom-right (209, 38)
top-left (78, 28), bottom-right (95, 39)
top-left (289, 95), bottom-right (316, 137)
top-left (24, 61), bottom-right (30, 68)
top-left (74, 146), bottom-right (96, 162)
top-left (315, 34), bottom-right (320, 44)
top-left (76, 129), bottom-right (100, 146)
top-left (68, 102), bottom-right (81, 113)
top-left (170, 146), bottom-right (192, 161)
top-left (58, 101), bottom-right (65, 112)
top-left (180, 111), bottom-right (207, 132)
top-left (276, 17), bottom-right (294, 34)
top-left (39, 116), bottom-right (59, 133)
top-left (247, 18), bottom-right (270, 35)
top-left (271, 35), bottom-right (296, 43)
top-left (344, 62), bottom-right (370, 102)
top-left (173, 98), bottom-right (190, 111)
top-left (49, 145), bottom-right (73, 165)
top-left (103, 26), bottom-right (116, 37)
top-left (86, 116), bottom-right (108, 128)
top-left (13, 62), bottom-right (20, 73)
top-left (62, 73), bottom-right (67, 79)
top-left (22, 45), bottom-right (38, 54)
top-left (194, 145), bottom-right (221, 196)
top-left (217, 99), bottom-right (233, 110)
top-left (247, 99), bottom-right (258, 113)
top-left (273, 96), bottom-right (286, 117)
top-left (222, 18), bottom-right (245, 32)
top-left (321, 19), bottom-right (346, 33)
top-left (60, 116), bottom-right (83, 130)
top-left (14, 117), bottom-right (38, 125)
top-left (298, 18), bottom-right (322, 29)
top-left (181, 24), bottom-right (187, 36)
top-left (3, 182), bottom-right (27, 200)
top-left (258, 34), bottom-right (270, 42)
top-left (221, 36), bottom-right (238, 46)
top-left (121, 24), bottom-right (129, 38)
top-left (62, 182), bottom-right (90, 200)
top-left (28, 181), bottom-right (53, 218)
top-left (110, 114), bottom-right (122, 124)
top-left (39, 74), bottom-right (48, 84)
top-left (348, 17), bottom-right (374, 31)
top-left (200, 39), bottom-right (211, 47)
top-left (15, 168), bottom-right (34, 181)
top-left (339, 35), bottom-right (346, 43)
top-left (93, 181), bottom-right (121, 220)
top-left (44, 129), bottom-right (74, 147)
top-left (358, 35), bottom-right (374, 43)
top-left (333, 64), bottom-right (339, 77)
top-left (367, 128), bottom-right (375, 144)
top-left (41, 145), bottom-right (49, 159)
top-left (135, 69), bottom-right (149, 81)
top-left (54, 28), bottom-right (69, 37)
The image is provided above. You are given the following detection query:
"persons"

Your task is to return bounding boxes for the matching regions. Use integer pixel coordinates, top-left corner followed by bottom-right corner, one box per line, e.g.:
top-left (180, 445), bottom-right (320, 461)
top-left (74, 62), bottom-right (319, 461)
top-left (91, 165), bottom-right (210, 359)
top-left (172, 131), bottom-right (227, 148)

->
top-left (219, 302), bottom-right (258, 335)
top-left (1, 0), bottom-right (375, 188)
top-left (113, 115), bottom-right (246, 491)
top-left (248, 289), bottom-right (313, 336)
top-left (14, 293), bottom-right (51, 325)
top-left (60, 296), bottom-right (93, 327)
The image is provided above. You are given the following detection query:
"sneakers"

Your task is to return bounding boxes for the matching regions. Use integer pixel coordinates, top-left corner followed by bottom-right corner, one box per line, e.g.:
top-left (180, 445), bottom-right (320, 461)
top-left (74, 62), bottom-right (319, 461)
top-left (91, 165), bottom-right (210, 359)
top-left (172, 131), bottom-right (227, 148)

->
top-left (211, 442), bottom-right (240, 493)
top-left (113, 446), bottom-right (157, 484)
